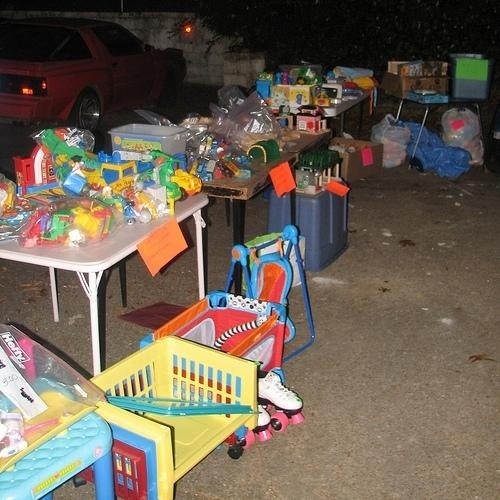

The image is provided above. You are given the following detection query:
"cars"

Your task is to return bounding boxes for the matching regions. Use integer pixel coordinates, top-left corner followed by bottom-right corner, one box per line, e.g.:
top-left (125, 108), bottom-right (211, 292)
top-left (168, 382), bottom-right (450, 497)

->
top-left (0, 15), bottom-right (184, 137)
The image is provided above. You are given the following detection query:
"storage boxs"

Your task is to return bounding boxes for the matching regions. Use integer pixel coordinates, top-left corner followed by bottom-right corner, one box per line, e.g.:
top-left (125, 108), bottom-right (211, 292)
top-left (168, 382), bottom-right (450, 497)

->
top-left (328, 136), bottom-right (383, 184)
top-left (380, 53), bottom-right (491, 100)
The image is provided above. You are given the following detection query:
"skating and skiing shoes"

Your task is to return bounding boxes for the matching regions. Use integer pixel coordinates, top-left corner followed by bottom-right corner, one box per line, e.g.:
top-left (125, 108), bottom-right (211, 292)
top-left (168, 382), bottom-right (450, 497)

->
top-left (240, 403), bottom-right (271, 449)
top-left (257, 370), bottom-right (305, 432)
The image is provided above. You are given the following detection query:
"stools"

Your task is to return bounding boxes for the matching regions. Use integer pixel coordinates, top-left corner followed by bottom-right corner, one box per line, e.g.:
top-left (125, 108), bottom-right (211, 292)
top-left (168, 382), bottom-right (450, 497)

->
top-left (393, 98), bottom-right (487, 171)
top-left (0, 412), bottom-right (114, 499)
